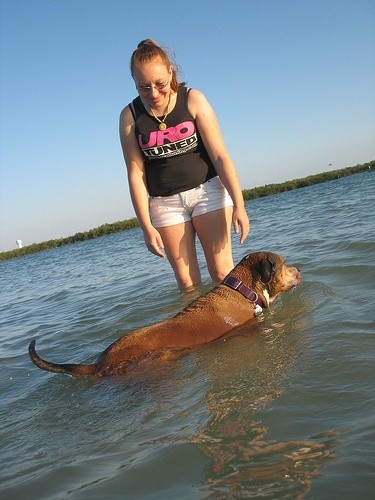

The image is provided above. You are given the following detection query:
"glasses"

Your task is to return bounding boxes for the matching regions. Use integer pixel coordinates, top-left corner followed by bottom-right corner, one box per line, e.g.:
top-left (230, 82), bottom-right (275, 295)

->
top-left (137, 78), bottom-right (170, 92)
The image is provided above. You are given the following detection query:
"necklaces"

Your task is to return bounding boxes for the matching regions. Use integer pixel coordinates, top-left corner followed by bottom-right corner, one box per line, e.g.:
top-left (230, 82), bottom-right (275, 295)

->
top-left (149, 91), bottom-right (172, 132)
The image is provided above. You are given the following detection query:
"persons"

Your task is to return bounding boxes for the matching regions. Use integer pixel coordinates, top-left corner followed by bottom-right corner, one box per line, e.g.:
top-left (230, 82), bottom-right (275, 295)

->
top-left (119, 39), bottom-right (250, 294)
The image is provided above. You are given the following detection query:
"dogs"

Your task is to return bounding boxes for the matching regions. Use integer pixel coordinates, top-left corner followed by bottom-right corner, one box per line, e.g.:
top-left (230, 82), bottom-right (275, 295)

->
top-left (28, 250), bottom-right (302, 380)
